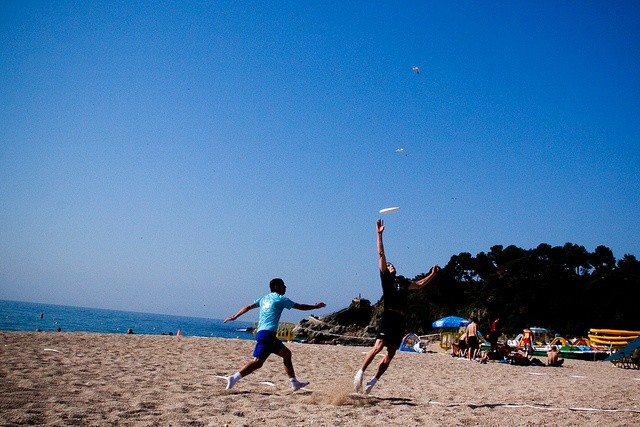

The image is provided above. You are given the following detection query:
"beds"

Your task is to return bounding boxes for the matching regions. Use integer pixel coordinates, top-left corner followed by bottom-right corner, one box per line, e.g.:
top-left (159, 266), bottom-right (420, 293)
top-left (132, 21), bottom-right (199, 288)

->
top-left (432, 315), bottom-right (471, 349)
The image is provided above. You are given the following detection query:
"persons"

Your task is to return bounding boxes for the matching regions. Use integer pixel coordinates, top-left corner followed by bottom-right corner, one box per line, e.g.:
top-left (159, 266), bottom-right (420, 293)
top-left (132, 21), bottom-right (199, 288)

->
top-left (522, 327), bottom-right (533, 356)
top-left (128, 328), bottom-right (135, 335)
top-left (489, 318), bottom-right (500, 349)
top-left (224, 277), bottom-right (326, 391)
top-left (465, 317), bottom-right (479, 359)
top-left (354, 219), bottom-right (438, 393)
top-left (548, 345), bottom-right (564, 365)
top-left (457, 332), bottom-right (468, 358)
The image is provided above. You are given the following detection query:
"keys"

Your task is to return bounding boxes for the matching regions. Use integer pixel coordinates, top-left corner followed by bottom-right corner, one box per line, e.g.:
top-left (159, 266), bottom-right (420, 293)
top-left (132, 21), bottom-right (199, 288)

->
top-left (379, 207), bottom-right (400, 213)
